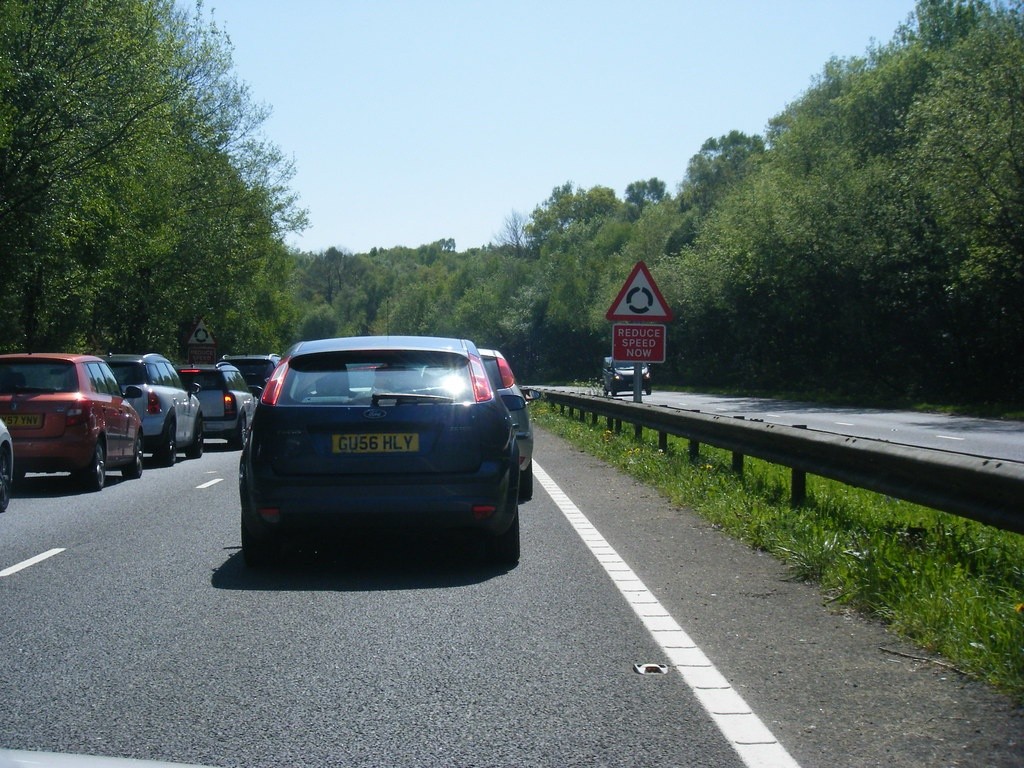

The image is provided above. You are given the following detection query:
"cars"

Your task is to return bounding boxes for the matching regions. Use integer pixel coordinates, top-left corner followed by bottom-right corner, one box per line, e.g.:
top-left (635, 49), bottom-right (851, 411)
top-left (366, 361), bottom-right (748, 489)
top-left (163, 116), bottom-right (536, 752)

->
top-left (0, 419), bottom-right (13, 514)
top-left (237, 335), bottom-right (520, 562)
top-left (475, 348), bottom-right (542, 504)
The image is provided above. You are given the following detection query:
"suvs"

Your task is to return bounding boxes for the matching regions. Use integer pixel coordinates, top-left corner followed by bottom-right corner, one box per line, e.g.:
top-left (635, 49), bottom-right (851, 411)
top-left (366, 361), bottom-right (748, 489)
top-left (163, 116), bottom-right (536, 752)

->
top-left (0, 353), bottom-right (143, 492)
top-left (95, 352), bottom-right (204, 467)
top-left (175, 361), bottom-right (263, 450)
top-left (221, 354), bottom-right (286, 398)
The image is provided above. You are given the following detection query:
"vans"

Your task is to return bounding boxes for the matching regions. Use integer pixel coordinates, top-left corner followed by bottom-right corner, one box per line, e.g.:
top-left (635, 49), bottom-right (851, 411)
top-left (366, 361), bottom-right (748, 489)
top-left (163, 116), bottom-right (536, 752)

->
top-left (602, 355), bottom-right (651, 395)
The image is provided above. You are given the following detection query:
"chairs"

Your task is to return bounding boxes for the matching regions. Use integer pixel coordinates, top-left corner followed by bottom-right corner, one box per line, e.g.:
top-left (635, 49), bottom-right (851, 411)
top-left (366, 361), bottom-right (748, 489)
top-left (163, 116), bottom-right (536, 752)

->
top-left (312, 364), bottom-right (358, 398)
